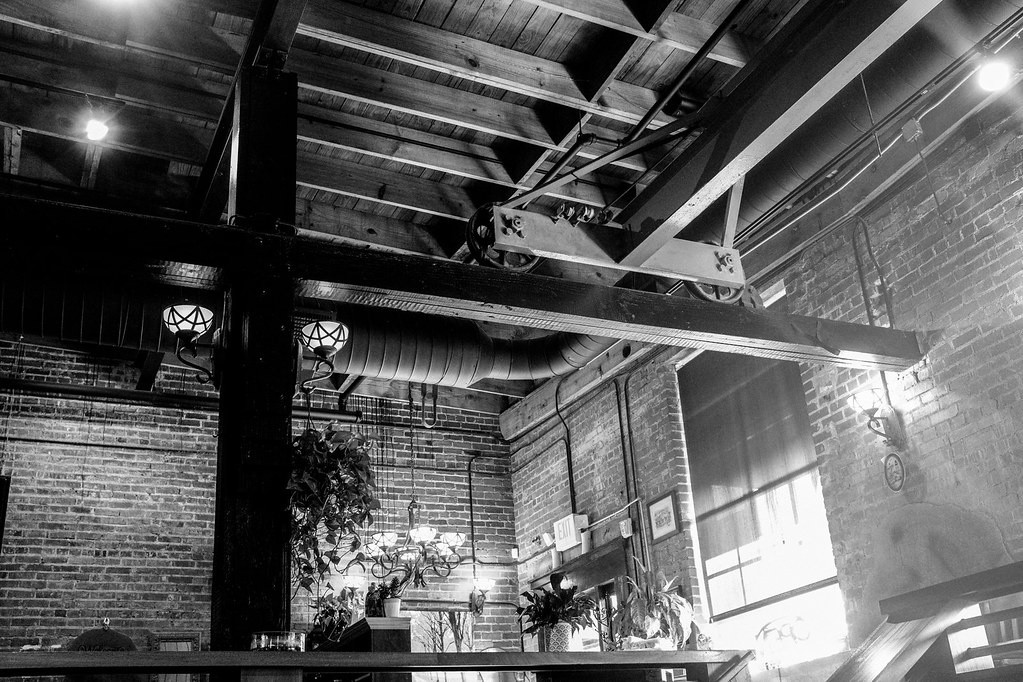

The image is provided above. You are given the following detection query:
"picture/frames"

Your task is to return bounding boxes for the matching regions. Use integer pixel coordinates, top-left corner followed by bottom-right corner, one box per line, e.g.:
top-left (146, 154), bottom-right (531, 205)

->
top-left (646, 489), bottom-right (682, 546)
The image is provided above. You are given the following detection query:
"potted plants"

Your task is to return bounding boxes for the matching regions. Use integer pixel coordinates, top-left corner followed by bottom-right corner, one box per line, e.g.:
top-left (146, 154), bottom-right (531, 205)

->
top-left (846, 388), bottom-right (906, 450)
top-left (514, 569), bottom-right (598, 653)
top-left (365, 577), bottom-right (401, 618)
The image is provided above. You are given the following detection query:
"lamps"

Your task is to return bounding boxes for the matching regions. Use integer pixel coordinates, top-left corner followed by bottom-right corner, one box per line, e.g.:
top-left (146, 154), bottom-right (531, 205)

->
top-left (362, 383), bottom-right (466, 587)
top-left (294, 321), bottom-right (349, 395)
top-left (164, 305), bottom-right (220, 392)
